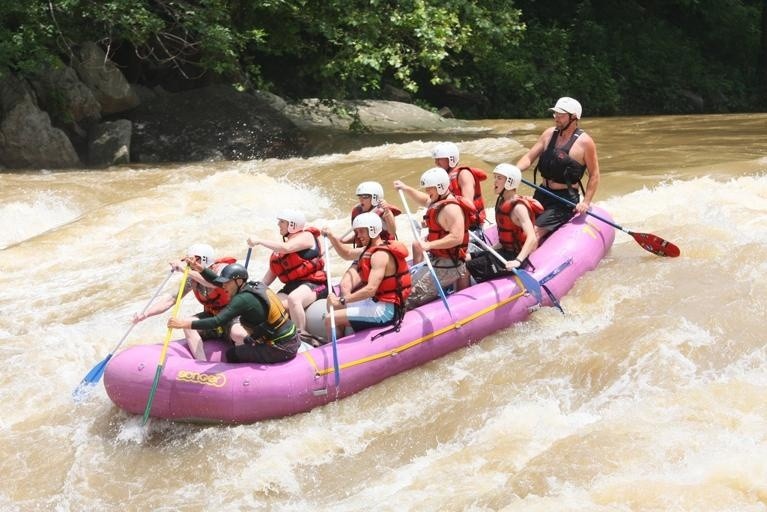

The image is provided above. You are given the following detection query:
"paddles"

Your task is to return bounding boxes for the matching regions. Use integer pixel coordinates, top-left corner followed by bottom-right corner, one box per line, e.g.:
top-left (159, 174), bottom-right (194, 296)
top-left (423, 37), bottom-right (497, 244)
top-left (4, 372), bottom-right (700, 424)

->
top-left (323, 232), bottom-right (340, 387)
top-left (398, 189), bottom-right (453, 319)
top-left (469, 230), bottom-right (542, 303)
top-left (74, 268), bottom-right (175, 395)
top-left (142, 263), bottom-right (191, 425)
top-left (521, 178), bottom-right (680, 257)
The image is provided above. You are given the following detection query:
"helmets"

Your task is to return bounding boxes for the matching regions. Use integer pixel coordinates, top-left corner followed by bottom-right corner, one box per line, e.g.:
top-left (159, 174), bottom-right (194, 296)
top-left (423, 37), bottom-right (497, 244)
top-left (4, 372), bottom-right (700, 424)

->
top-left (357, 181), bottom-right (385, 207)
top-left (213, 263), bottom-right (248, 283)
top-left (492, 162), bottom-right (522, 191)
top-left (419, 168), bottom-right (450, 196)
top-left (186, 244), bottom-right (214, 267)
top-left (277, 209), bottom-right (305, 233)
top-left (433, 141), bottom-right (460, 168)
top-left (353, 212), bottom-right (383, 239)
top-left (548, 96), bottom-right (582, 121)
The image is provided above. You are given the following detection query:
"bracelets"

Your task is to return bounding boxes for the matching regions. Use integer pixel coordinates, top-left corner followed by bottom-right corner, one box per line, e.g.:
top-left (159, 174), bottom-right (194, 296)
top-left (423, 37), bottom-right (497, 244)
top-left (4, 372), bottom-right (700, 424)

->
top-left (339, 297), bottom-right (346, 306)
top-left (515, 258), bottom-right (523, 264)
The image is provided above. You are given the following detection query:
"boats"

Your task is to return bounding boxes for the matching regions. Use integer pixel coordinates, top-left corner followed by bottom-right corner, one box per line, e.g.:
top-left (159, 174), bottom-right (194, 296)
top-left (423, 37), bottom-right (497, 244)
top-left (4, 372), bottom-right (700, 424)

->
top-left (97, 198), bottom-right (619, 428)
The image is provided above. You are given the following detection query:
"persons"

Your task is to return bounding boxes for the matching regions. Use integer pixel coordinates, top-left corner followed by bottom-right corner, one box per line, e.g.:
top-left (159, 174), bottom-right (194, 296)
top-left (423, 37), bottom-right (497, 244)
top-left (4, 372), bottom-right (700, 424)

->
top-left (130, 244), bottom-right (248, 361)
top-left (245, 204), bottom-right (331, 332)
top-left (455, 162), bottom-right (545, 291)
top-left (334, 180), bottom-right (403, 298)
top-left (393, 166), bottom-right (471, 311)
top-left (409, 140), bottom-right (487, 266)
top-left (318, 211), bottom-right (413, 344)
top-left (512, 95), bottom-right (600, 253)
top-left (166, 263), bottom-right (302, 364)
